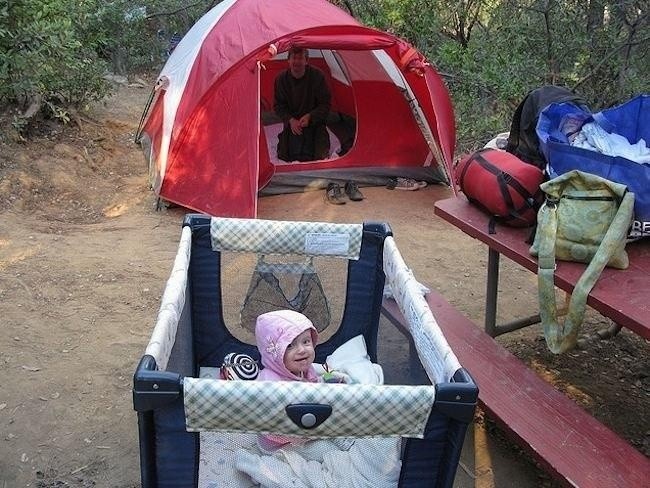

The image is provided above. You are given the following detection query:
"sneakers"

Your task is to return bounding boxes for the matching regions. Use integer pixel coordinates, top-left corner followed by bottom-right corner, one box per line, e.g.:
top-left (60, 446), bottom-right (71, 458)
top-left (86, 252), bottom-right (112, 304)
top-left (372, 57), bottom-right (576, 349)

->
top-left (326, 182), bottom-right (364, 204)
top-left (386, 177), bottom-right (427, 191)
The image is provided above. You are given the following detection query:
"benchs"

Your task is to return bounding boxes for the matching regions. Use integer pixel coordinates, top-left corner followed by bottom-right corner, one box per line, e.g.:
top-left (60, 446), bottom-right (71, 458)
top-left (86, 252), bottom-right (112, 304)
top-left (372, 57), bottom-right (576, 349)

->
top-left (382, 284), bottom-right (648, 486)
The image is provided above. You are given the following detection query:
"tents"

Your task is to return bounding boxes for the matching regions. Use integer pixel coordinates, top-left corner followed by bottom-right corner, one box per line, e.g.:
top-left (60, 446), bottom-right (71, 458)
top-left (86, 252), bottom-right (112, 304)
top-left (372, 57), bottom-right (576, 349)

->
top-left (134, 0), bottom-right (458, 219)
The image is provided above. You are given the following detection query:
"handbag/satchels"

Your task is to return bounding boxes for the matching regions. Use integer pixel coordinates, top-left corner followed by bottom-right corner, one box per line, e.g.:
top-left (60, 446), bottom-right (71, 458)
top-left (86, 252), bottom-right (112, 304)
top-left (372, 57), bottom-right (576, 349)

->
top-left (528, 169), bottom-right (635, 270)
top-left (276, 125), bottom-right (330, 162)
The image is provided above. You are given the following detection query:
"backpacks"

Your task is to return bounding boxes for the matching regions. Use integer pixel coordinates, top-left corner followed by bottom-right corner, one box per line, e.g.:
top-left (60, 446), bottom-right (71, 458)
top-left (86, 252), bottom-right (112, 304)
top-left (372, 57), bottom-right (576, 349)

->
top-left (505, 85), bottom-right (591, 182)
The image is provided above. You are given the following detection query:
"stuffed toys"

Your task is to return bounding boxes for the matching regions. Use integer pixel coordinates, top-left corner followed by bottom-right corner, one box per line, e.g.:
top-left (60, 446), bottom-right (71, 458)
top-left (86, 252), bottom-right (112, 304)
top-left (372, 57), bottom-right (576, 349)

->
top-left (219, 352), bottom-right (259, 382)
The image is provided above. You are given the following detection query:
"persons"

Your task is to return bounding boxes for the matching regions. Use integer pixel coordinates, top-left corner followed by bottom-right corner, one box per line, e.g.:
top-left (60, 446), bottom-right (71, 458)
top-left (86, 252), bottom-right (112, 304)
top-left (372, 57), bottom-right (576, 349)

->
top-left (272, 47), bottom-right (331, 162)
top-left (255, 309), bottom-right (358, 457)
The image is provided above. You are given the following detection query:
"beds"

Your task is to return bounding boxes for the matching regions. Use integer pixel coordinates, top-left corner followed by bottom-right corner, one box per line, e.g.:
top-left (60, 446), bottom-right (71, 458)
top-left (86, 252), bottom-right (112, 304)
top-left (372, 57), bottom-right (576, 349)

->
top-left (131, 213), bottom-right (478, 486)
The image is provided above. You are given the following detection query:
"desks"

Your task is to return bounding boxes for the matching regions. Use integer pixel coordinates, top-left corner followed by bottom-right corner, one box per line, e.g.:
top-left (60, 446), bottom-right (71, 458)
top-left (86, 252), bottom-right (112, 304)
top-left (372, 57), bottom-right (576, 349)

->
top-left (436, 186), bottom-right (649, 340)
